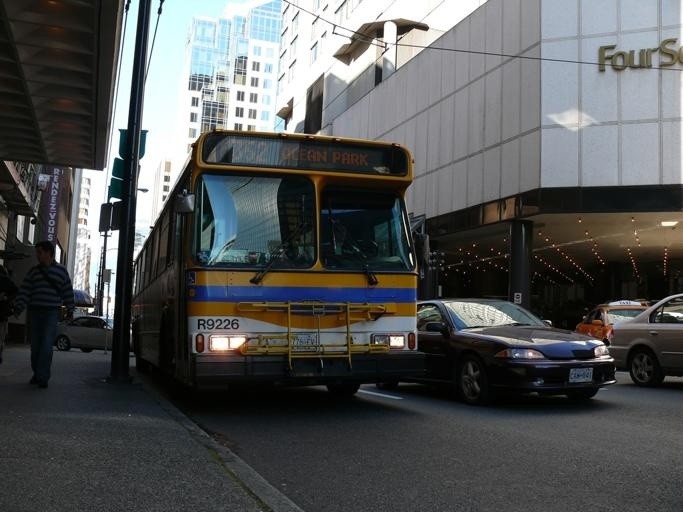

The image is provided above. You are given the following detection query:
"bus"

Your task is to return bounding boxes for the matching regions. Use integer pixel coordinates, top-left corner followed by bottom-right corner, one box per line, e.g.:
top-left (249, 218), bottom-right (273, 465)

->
top-left (132, 129), bottom-right (420, 398)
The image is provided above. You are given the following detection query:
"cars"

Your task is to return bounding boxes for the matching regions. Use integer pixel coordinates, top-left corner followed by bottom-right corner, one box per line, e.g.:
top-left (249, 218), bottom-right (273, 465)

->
top-left (53, 314), bottom-right (116, 354)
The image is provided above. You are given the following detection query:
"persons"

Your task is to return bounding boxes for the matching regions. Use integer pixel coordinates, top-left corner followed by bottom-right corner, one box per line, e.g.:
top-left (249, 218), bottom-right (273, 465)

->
top-left (0, 265), bottom-right (19, 363)
top-left (14, 240), bottom-right (75, 389)
top-left (322, 222), bottom-right (378, 257)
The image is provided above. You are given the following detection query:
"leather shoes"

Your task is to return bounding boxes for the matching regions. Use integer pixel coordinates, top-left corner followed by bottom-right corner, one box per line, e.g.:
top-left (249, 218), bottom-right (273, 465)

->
top-left (30, 375), bottom-right (51, 388)
